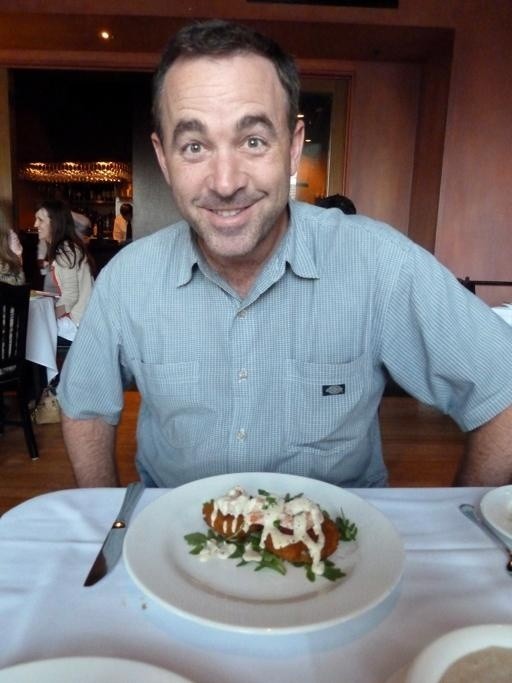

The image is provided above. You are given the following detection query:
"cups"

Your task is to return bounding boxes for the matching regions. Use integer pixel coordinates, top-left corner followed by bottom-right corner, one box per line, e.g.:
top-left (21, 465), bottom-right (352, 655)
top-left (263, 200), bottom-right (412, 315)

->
top-left (400, 622), bottom-right (512, 682)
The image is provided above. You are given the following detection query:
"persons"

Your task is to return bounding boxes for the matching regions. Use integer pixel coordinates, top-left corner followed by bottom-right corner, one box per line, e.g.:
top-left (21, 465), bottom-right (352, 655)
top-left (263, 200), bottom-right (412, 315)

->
top-left (0, 214), bottom-right (26, 359)
top-left (120, 204), bottom-right (133, 243)
top-left (52, 19), bottom-right (511, 490)
top-left (26, 202), bottom-right (95, 411)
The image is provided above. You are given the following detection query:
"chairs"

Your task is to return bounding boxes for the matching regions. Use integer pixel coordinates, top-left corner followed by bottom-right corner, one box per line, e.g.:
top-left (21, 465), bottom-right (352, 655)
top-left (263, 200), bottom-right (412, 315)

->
top-left (0, 289), bottom-right (40, 461)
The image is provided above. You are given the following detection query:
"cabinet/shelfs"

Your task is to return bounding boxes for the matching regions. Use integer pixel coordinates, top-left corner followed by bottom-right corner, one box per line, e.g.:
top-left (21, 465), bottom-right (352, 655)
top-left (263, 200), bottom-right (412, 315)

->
top-left (70, 199), bottom-right (115, 239)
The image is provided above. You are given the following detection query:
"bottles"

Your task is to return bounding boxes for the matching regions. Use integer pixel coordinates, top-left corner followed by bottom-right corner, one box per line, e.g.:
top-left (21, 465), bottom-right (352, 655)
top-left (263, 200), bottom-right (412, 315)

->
top-left (34, 186), bottom-right (133, 241)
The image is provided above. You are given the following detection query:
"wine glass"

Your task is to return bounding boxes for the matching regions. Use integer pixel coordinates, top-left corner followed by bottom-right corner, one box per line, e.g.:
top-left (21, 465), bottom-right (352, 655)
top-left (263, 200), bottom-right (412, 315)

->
top-left (13, 159), bottom-right (132, 184)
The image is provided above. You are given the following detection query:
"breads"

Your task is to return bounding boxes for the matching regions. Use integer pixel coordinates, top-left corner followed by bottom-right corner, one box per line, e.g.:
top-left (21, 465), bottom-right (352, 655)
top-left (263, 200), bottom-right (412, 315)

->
top-left (200, 485), bottom-right (341, 563)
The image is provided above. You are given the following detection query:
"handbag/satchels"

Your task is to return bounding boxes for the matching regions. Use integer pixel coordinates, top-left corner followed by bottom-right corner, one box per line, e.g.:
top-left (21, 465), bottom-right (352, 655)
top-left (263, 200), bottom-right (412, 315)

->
top-left (30, 388), bottom-right (61, 426)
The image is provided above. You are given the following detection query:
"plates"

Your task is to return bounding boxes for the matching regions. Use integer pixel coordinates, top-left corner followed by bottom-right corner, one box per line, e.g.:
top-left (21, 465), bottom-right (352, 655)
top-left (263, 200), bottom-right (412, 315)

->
top-left (125, 472), bottom-right (410, 637)
top-left (2, 656), bottom-right (197, 683)
top-left (478, 485), bottom-right (511, 555)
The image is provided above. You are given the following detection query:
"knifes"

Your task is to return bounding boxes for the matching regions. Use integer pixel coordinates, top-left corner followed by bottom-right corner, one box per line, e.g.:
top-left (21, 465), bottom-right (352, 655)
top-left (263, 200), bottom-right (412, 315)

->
top-left (83, 476), bottom-right (146, 588)
